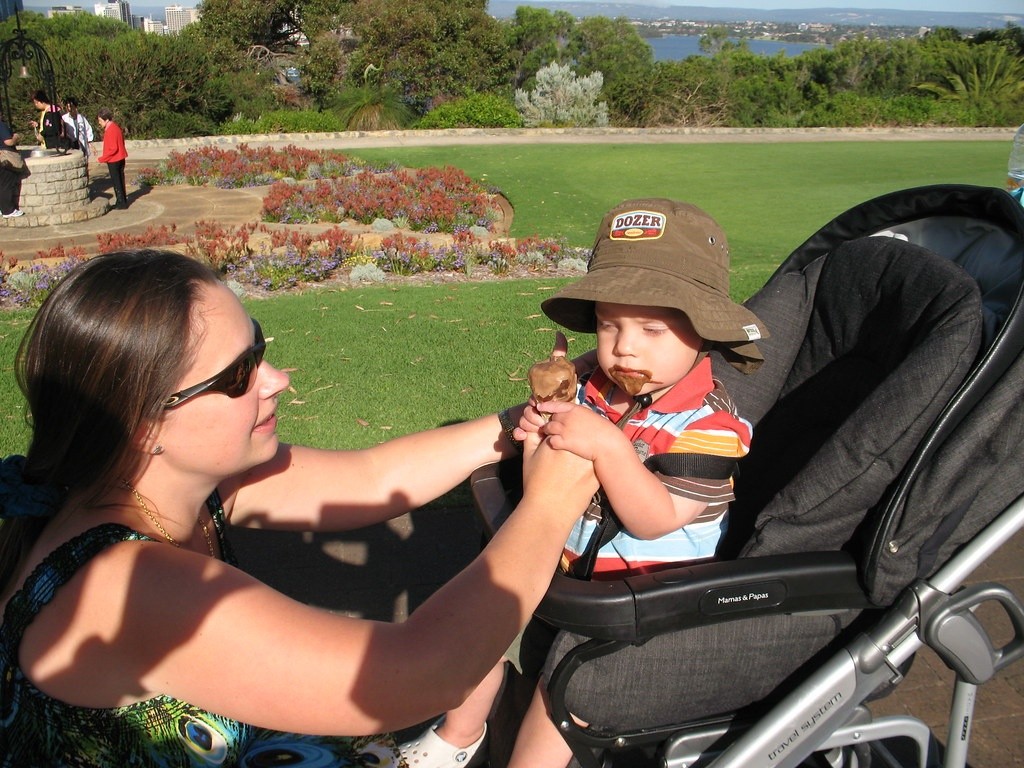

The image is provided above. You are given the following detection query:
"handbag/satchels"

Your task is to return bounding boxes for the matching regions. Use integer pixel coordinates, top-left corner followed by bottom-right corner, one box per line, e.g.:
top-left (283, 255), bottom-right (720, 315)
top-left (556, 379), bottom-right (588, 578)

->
top-left (54, 134), bottom-right (79, 153)
top-left (0, 149), bottom-right (26, 173)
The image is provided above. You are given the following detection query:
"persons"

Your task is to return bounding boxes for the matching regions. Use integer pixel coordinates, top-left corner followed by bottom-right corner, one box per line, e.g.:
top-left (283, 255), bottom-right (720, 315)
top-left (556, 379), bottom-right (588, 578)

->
top-left (30, 91), bottom-right (70, 149)
top-left (352, 201), bottom-right (768, 768)
top-left (0, 250), bottom-right (601, 768)
top-left (0, 102), bottom-right (23, 218)
top-left (95, 112), bottom-right (129, 209)
top-left (62, 98), bottom-right (98, 156)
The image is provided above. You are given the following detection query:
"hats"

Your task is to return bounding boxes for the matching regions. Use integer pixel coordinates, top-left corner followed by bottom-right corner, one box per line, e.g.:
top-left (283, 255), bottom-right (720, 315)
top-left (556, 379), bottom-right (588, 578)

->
top-left (540, 199), bottom-right (770, 374)
top-left (34, 90), bottom-right (49, 103)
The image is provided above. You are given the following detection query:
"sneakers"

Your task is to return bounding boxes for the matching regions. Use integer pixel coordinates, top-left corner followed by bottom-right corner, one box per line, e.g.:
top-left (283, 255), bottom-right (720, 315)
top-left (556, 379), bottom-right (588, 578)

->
top-left (0, 209), bottom-right (24, 218)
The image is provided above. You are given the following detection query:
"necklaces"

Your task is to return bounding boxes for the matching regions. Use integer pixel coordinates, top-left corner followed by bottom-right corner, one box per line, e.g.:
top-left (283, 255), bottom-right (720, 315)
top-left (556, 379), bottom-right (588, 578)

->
top-left (122, 479), bottom-right (214, 559)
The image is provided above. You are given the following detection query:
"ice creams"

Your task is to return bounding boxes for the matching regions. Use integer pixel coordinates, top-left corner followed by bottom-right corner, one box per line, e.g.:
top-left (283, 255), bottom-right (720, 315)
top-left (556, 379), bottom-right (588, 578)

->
top-left (528, 356), bottom-right (577, 424)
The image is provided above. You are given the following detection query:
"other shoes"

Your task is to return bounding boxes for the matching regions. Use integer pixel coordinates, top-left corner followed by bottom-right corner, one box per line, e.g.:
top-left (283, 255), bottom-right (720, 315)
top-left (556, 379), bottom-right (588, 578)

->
top-left (111, 205), bottom-right (127, 209)
top-left (399, 721), bottom-right (489, 768)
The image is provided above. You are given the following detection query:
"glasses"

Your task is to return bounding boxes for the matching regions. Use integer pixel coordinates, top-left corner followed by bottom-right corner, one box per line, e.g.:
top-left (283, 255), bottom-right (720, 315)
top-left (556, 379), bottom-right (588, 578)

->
top-left (158, 317), bottom-right (267, 408)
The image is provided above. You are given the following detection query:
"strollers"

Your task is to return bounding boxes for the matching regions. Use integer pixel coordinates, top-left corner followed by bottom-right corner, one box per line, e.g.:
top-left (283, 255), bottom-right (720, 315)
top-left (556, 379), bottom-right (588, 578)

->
top-left (417, 127), bottom-right (1024, 768)
